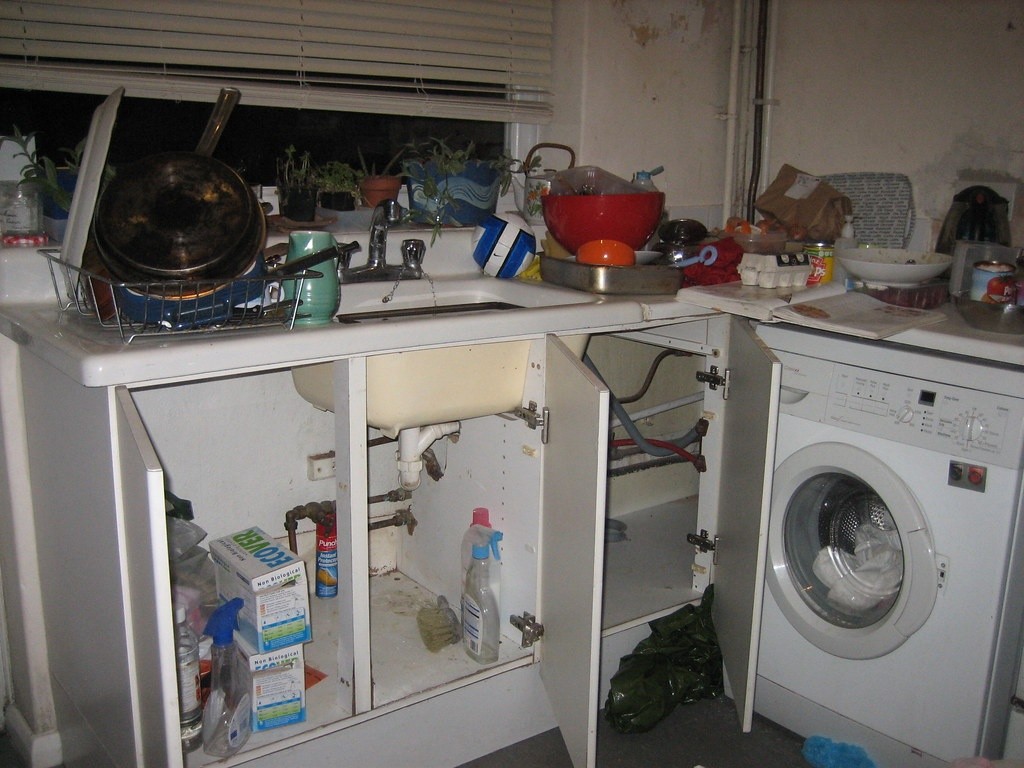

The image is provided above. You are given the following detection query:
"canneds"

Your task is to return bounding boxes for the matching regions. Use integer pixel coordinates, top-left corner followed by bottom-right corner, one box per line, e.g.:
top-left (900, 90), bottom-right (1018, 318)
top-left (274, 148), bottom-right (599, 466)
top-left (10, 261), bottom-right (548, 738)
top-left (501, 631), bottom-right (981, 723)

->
top-left (803, 242), bottom-right (835, 286)
top-left (970, 261), bottom-right (1016, 306)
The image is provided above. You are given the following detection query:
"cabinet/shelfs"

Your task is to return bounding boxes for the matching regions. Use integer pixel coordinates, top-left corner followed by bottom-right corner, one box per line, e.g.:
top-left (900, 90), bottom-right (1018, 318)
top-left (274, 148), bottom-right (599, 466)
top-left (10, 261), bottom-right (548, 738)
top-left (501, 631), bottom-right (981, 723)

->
top-left (17, 305), bottom-right (784, 768)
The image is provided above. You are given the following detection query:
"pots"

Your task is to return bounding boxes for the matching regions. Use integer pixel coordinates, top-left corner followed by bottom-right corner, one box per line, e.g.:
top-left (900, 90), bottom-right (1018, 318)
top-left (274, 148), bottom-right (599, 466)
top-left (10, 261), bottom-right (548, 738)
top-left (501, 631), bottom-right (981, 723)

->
top-left (95, 85), bottom-right (265, 301)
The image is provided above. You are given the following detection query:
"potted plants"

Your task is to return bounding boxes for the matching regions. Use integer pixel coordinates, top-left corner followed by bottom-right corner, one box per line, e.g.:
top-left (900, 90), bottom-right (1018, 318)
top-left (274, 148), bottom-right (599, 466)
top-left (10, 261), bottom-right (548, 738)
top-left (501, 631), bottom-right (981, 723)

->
top-left (395, 134), bottom-right (542, 248)
top-left (316, 160), bottom-right (358, 211)
top-left (330, 143), bottom-right (409, 207)
top-left (275, 142), bottom-right (321, 221)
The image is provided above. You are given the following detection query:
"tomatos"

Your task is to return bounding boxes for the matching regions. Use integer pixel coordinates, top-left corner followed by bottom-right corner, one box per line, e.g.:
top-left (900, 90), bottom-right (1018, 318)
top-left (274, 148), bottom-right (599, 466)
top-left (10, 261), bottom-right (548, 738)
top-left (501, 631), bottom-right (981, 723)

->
top-left (987, 274), bottom-right (1016, 295)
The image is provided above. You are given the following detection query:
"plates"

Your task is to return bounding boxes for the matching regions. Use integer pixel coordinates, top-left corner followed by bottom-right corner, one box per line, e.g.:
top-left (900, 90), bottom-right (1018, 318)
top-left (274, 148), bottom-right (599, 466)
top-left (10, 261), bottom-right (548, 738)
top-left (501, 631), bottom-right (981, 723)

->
top-left (566, 251), bottom-right (663, 265)
top-left (835, 247), bottom-right (952, 286)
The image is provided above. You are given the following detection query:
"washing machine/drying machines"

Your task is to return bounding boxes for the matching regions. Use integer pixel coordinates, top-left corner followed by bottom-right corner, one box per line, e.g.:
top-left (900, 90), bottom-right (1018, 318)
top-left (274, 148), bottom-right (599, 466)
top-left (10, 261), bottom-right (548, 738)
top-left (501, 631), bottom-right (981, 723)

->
top-left (720, 321), bottom-right (1023, 768)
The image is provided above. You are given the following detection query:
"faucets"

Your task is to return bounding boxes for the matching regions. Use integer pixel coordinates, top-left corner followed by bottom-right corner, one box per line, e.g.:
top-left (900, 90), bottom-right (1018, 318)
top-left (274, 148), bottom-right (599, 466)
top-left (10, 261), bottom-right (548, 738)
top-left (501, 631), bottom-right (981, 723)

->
top-left (366, 198), bottom-right (401, 268)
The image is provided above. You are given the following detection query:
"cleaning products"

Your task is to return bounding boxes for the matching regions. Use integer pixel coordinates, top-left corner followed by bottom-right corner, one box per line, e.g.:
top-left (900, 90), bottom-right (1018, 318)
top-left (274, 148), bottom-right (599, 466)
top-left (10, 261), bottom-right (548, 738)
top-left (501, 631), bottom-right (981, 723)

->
top-left (200, 596), bottom-right (257, 759)
top-left (457, 506), bottom-right (506, 667)
top-left (833, 213), bottom-right (860, 282)
top-left (631, 165), bottom-right (666, 253)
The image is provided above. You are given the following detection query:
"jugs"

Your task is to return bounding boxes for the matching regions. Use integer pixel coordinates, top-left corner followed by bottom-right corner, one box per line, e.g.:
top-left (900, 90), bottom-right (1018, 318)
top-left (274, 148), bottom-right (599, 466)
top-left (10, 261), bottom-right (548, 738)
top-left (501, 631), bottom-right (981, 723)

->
top-left (283, 229), bottom-right (340, 324)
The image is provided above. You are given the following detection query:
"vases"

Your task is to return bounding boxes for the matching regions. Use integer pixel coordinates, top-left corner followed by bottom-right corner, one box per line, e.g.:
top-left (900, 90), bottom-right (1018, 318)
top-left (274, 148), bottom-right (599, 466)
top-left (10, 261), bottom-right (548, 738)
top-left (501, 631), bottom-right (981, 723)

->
top-left (281, 230), bottom-right (341, 325)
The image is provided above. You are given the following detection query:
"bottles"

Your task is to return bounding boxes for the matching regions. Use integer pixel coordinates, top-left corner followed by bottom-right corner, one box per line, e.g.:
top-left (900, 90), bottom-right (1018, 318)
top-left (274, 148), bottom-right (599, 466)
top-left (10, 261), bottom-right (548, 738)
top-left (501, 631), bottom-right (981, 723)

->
top-left (174, 604), bottom-right (203, 754)
top-left (462, 508), bottom-right (501, 604)
top-left (632, 172), bottom-right (659, 193)
top-left (833, 215), bottom-right (857, 284)
top-left (0, 179), bottom-right (44, 235)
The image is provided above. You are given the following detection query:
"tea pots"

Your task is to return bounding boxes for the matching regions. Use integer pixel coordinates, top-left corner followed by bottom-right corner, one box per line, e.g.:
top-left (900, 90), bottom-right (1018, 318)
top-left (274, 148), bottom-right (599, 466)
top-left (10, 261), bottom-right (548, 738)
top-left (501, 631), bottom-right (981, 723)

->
top-left (511, 143), bottom-right (576, 225)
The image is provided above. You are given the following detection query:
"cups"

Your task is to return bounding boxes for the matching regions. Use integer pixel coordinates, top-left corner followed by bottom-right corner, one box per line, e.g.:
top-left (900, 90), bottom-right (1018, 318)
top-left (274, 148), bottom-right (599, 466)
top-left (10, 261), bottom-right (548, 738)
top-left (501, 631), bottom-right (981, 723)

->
top-left (972, 261), bottom-right (1017, 304)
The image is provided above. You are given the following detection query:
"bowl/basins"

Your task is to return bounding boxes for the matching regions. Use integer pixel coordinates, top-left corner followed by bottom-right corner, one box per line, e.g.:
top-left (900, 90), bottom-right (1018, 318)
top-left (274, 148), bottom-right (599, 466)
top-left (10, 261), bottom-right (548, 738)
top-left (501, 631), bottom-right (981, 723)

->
top-left (719, 231), bottom-right (788, 254)
top-left (577, 240), bottom-right (635, 266)
top-left (957, 295), bottom-right (1024, 334)
top-left (541, 191), bottom-right (664, 255)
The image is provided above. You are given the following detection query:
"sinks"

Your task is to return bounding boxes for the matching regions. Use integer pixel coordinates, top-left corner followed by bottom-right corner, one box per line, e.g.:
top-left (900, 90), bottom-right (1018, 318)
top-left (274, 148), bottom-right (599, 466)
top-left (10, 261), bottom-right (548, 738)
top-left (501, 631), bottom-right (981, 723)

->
top-left (290, 278), bottom-right (644, 443)
